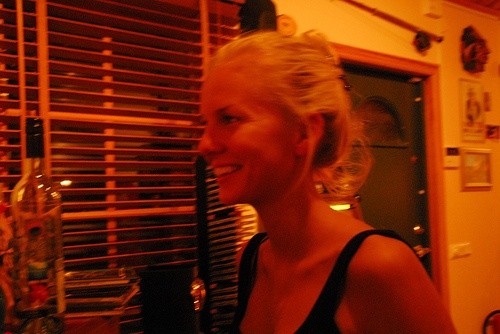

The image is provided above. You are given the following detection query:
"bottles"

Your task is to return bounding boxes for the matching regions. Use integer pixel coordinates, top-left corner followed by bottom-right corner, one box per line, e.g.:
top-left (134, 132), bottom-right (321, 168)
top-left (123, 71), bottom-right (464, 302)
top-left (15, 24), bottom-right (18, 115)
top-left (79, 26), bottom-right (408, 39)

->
top-left (10, 118), bottom-right (65, 318)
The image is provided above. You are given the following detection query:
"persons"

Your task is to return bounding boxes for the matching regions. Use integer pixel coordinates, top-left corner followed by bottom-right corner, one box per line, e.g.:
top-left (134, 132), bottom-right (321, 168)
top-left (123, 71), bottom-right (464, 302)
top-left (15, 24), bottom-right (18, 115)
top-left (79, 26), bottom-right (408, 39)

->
top-left (197, 33), bottom-right (458, 334)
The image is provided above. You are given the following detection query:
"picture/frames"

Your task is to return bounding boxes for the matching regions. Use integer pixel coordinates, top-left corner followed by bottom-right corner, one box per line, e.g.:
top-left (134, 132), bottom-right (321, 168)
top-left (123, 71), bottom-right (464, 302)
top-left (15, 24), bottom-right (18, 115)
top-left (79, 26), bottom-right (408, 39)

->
top-left (458, 75), bottom-right (487, 132)
top-left (460, 147), bottom-right (494, 190)
